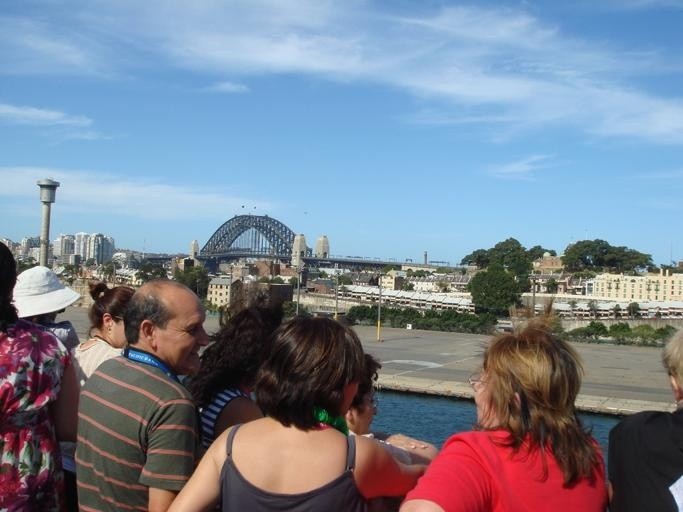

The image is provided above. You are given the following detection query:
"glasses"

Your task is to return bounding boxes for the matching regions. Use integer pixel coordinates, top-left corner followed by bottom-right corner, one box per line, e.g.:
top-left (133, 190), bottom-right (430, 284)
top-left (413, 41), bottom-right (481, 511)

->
top-left (360, 397), bottom-right (379, 408)
top-left (467, 374), bottom-right (489, 386)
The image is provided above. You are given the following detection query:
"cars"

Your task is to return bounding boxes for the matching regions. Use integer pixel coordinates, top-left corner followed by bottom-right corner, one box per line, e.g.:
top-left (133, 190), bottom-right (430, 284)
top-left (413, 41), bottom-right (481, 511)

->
top-left (374, 270), bottom-right (383, 342)
top-left (332, 269), bottom-right (341, 321)
top-left (292, 265), bottom-right (304, 318)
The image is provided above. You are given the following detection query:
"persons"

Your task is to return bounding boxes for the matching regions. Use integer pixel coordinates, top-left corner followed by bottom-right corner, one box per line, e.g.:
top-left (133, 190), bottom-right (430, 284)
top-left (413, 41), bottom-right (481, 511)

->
top-left (10, 265), bottom-right (87, 350)
top-left (75, 279), bottom-right (209, 512)
top-left (165, 317), bottom-right (432, 512)
top-left (52, 282), bottom-right (136, 512)
top-left (607, 329), bottom-right (683, 512)
top-left (398, 315), bottom-right (607, 512)
top-left (0, 243), bottom-right (72, 512)
top-left (188, 306), bottom-right (286, 455)
top-left (345, 354), bottom-right (382, 438)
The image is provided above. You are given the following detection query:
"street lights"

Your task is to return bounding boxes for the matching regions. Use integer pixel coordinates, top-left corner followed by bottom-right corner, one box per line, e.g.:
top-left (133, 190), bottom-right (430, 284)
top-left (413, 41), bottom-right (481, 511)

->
top-left (229, 264), bottom-right (234, 284)
top-left (33, 179), bottom-right (59, 268)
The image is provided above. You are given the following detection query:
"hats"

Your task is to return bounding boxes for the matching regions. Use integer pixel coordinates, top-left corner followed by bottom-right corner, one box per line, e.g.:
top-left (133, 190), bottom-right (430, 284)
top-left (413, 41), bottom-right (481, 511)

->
top-left (12, 265), bottom-right (82, 317)
